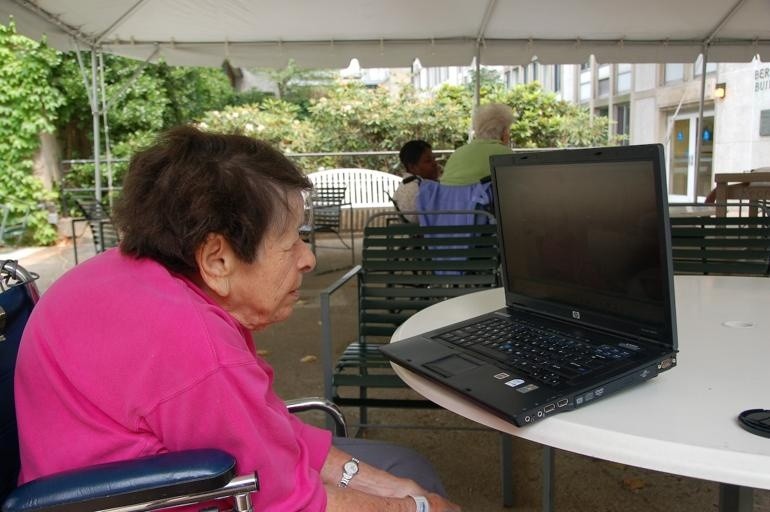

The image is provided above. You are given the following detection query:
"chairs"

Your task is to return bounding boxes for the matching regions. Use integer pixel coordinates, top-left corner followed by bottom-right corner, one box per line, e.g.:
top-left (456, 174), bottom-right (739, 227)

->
top-left (69, 195), bottom-right (118, 268)
top-left (298, 186), bottom-right (351, 252)
top-left (1, 260), bottom-right (353, 511)
top-left (319, 211), bottom-right (516, 510)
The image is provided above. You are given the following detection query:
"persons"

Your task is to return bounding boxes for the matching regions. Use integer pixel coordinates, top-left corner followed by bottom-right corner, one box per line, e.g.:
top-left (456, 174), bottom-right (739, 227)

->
top-left (13, 125), bottom-right (465, 511)
top-left (392, 139), bottom-right (437, 225)
top-left (440, 104), bottom-right (515, 185)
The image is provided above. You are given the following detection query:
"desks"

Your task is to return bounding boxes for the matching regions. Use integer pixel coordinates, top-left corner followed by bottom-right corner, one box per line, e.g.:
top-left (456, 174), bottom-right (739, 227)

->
top-left (391, 273), bottom-right (769, 512)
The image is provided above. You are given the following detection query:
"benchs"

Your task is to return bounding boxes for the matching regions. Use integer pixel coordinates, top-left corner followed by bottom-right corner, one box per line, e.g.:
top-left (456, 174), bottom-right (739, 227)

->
top-left (669, 215), bottom-right (770, 277)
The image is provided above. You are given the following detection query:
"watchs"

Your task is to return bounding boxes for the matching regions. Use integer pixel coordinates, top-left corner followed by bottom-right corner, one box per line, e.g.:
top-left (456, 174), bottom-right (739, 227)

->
top-left (337, 456), bottom-right (360, 488)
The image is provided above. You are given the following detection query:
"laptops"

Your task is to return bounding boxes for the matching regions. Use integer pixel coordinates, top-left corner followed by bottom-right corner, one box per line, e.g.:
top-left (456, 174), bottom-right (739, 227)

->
top-left (376, 143), bottom-right (679, 426)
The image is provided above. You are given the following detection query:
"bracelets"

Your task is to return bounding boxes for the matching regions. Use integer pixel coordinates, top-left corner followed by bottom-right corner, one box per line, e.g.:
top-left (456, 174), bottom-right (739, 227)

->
top-left (406, 495), bottom-right (429, 512)
